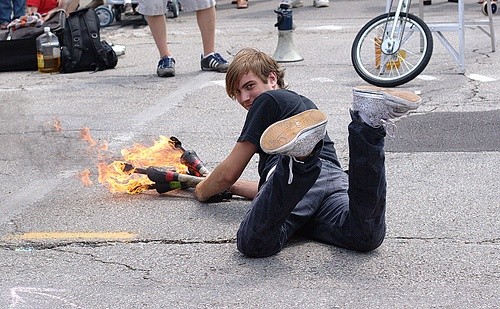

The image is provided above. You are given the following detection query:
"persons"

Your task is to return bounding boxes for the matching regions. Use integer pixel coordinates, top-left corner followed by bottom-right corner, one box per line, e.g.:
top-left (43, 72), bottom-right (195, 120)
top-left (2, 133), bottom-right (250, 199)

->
top-left (192, 47), bottom-right (423, 260)
top-left (135, 1), bottom-right (232, 78)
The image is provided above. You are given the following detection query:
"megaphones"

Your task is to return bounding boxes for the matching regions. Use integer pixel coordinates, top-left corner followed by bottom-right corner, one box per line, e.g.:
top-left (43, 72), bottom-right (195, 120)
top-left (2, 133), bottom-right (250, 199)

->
top-left (271, 3), bottom-right (303, 61)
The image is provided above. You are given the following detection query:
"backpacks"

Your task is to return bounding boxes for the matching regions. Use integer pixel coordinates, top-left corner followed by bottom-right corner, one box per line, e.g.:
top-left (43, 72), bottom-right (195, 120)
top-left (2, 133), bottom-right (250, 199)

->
top-left (58, 7), bottom-right (118, 74)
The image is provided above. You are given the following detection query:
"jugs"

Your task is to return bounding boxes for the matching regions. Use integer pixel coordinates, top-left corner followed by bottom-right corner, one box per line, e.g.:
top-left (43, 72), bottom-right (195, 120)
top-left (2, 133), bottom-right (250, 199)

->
top-left (36, 26), bottom-right (61, 74)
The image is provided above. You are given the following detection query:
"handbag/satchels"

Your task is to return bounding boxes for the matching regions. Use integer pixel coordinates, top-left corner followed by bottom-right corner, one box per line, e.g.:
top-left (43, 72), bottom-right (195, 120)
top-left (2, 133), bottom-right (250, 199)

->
top-left (0, 38), bottom-right (38, 72)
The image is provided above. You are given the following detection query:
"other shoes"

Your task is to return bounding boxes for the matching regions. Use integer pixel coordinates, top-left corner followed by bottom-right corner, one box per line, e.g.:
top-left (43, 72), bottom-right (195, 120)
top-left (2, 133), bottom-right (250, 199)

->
top-left (232, 0), bottom-right (249, 8)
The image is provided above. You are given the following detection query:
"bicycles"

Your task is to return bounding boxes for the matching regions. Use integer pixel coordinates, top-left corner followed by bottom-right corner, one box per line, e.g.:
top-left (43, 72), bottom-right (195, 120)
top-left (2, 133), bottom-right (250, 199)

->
top-left (349, 1), bottom-right (434, 87)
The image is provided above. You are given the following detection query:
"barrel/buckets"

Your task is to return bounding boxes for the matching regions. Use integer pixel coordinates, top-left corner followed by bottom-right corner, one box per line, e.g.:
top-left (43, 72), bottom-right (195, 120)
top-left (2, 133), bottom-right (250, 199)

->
top-left (374, 36), bottom-right (402, 70)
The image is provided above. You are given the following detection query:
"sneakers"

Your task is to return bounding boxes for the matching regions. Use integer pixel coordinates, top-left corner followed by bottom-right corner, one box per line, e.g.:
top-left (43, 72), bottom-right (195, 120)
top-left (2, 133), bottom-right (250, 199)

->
top-left (313, 0), bottom-right (330, 7)
top-left (157, 55), bottom-right (175, 77)
top-left (279, 0), bottom-right (304, 8)
top-left (352, 86), bottom-right (422, 141)
top-left (201, 53), bottom-right (230, 73)
top-left (260, 109), bottom-right (328, 185)
